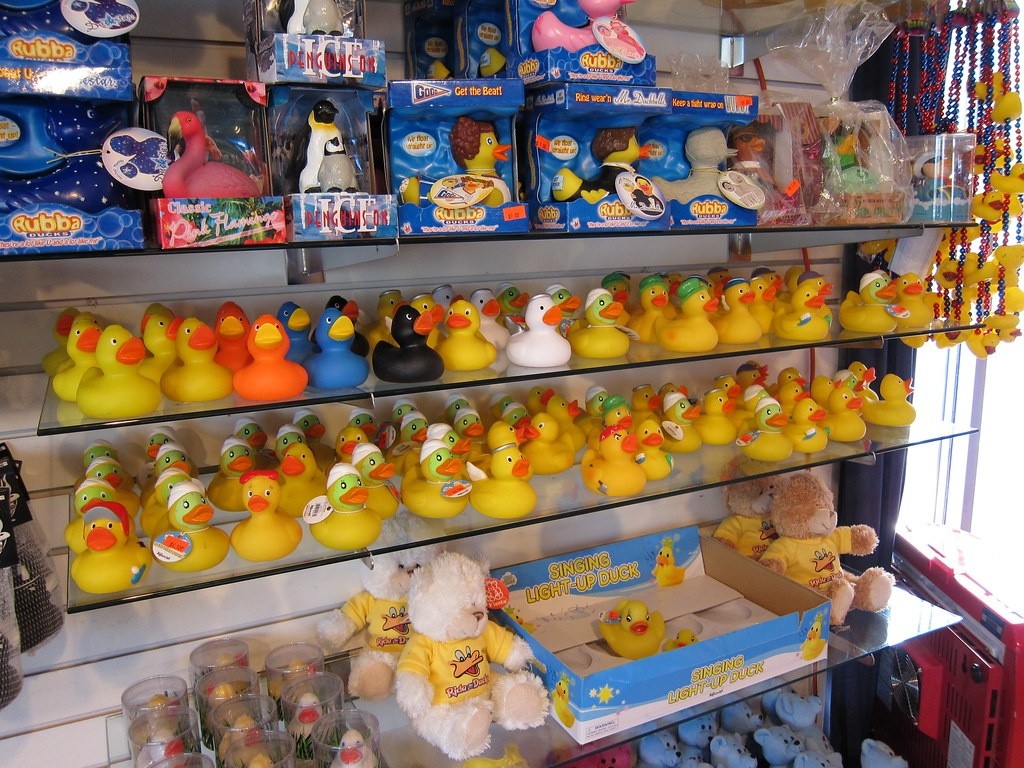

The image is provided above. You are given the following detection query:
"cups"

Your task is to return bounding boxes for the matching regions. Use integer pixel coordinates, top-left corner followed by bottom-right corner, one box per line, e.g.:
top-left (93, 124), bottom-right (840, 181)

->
top-left (121, 674), bottom-right (189, 731)
top-left (128, 707), bottom-right (201, 768)
top-left (212, 694), bottom-right (281, 768)
top-left (281, 671), bottom-right (345, 767)
top-left (265, 642), bottom-right (324, 722)
top-left (144, 752), bottom-right (215, 768)
top-left (189, 638), bottom-right (249, 713)
top-left (311, 709), bottom-right (381, 768)
top-left (196, 667), bottom-right (261, 752)
top-left (225, 730), bottom-right (296, 768)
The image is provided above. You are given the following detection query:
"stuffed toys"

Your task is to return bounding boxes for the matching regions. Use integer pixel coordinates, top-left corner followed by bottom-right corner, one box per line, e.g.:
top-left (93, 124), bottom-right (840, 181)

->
top-left (719, 695), bottom-right (764, 748)
top-left (546, 744), bottom-right (597, 768)
top-left (753, 723), bottom-right (801, 768)
top-left (679, 744), bottom-right (713, 768)
top-left (583, 737), bottom-right (632, 767)
top-left (775, 693), bottom-right (843, 768)
top-left (794, 747), bottom-right (842, 768)
top-left (315, 508), bottom-right (493, 703)
top-left (677, 708), bottom-right (717, 767)
top-left (759, 682), bottom-right (802, 726)
top-left (755, 471), bottom-right (897, 626)
top-left (711, 453), bottom-right (786, 560)
top-left (395, 550), bottom-right (552, 763)
top-left (860, 739), bottom-right (909, 768)
top-left (637, 722), bottom-right (684, 768)
top-left (709, 733), bottom-right (759, 768)
